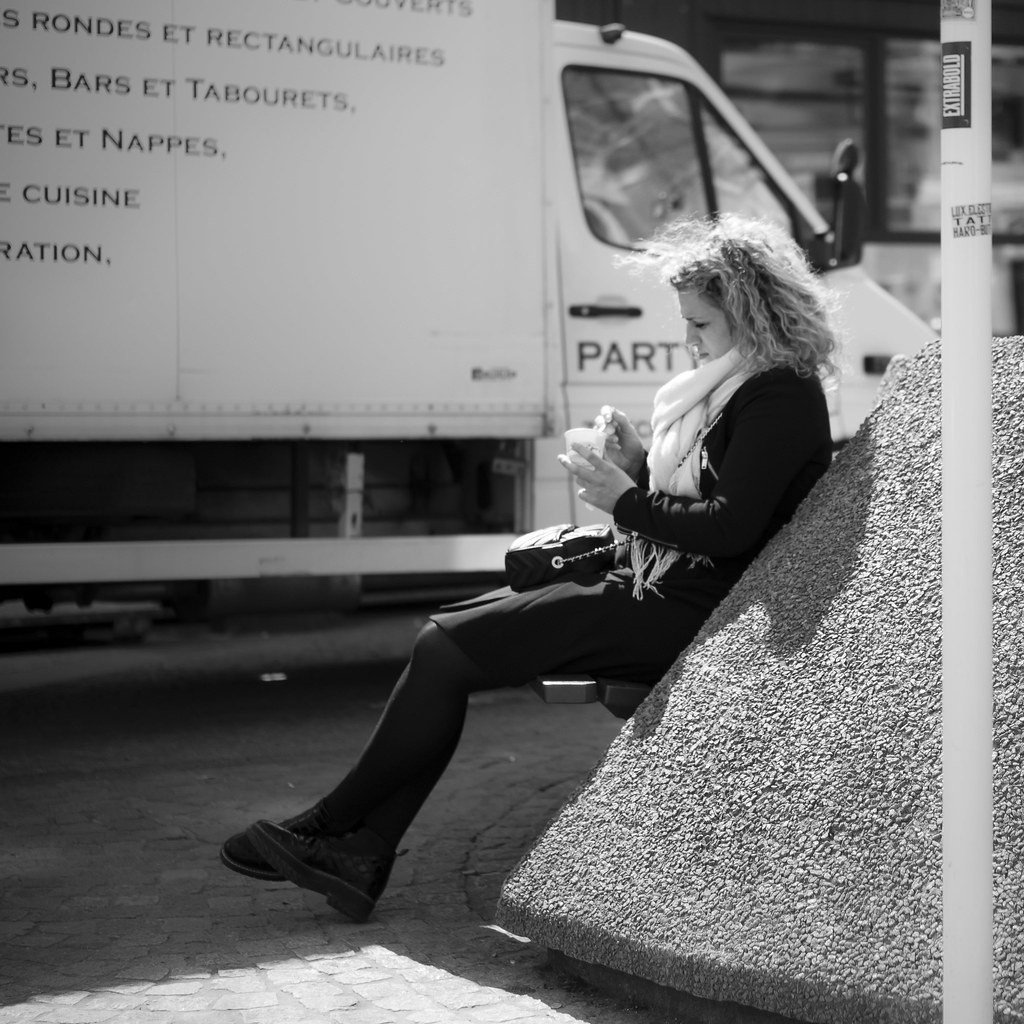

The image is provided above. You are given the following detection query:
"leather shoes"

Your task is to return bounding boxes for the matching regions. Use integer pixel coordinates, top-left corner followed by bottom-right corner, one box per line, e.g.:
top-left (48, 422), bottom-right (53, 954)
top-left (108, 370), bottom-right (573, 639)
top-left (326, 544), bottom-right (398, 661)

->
top-left (244, 817), bottom-right (398, 920)
top-left (219, 800), bottom-right (342, 884)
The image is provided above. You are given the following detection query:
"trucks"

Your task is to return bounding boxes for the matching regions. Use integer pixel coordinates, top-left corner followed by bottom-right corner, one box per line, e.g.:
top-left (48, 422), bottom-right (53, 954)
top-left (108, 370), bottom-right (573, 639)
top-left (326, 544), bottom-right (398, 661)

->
top-left (0, 0), bottom-right (939, 624)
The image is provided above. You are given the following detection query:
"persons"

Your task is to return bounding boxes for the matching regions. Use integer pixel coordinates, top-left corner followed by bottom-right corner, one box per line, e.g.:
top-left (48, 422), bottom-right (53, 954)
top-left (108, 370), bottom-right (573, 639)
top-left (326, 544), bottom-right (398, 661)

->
top-left (220, 209), bottom-right (836, 922)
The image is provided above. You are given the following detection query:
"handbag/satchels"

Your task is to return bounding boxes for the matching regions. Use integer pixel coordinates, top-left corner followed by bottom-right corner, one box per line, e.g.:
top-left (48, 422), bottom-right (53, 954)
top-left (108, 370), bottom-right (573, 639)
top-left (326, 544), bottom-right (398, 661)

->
top-left (502, 524), bottom-right (616, 595)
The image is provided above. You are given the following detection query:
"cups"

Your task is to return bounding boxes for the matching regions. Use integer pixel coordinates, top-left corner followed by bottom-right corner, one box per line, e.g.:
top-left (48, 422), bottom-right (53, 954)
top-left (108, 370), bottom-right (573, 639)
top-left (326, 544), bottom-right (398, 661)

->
top-left (564, 428), bottom-right (607, 472)
